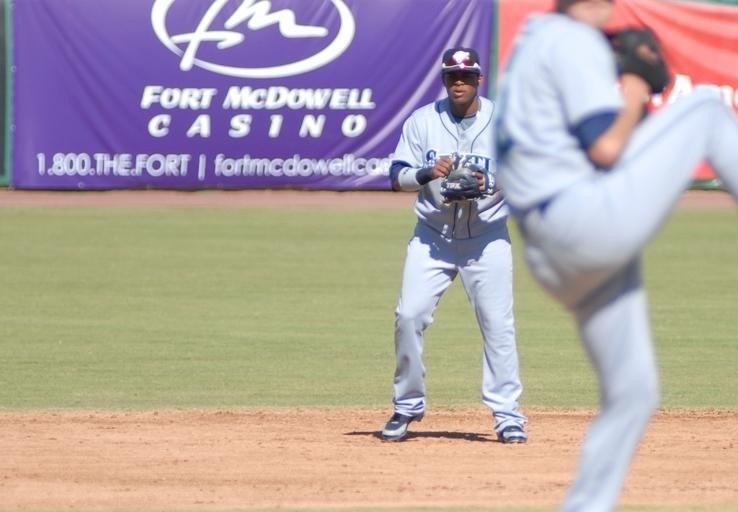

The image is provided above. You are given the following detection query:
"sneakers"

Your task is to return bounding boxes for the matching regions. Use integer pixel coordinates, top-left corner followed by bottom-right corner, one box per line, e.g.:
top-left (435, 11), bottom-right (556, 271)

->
top-left (496, 424), bottom-right (527, 443)
top-left (381, 413), bottom-right (425, 442)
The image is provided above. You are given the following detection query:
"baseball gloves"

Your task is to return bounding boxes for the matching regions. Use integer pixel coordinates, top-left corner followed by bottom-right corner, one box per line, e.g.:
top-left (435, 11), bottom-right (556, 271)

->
top-left (440, 164), bottom-right (497, 205)
top-left (610, 29), bottom-right (667, 92)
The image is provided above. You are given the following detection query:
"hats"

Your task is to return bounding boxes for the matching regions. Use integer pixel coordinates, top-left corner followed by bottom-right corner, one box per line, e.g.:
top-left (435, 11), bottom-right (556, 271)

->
top-left (442, 48), bottom-right (482, 75)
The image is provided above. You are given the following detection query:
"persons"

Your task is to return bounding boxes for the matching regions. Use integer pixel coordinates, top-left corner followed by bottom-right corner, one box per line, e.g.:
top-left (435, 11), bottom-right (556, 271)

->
top-left (494, 0), bottom-right (738, 512)
top-left (381, 46), bottom-right (529, 444)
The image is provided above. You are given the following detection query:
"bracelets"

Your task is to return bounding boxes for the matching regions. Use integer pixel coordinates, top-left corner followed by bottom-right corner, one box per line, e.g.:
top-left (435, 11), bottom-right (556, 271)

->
top-left (416, 167), bottom-right (434, 185)
